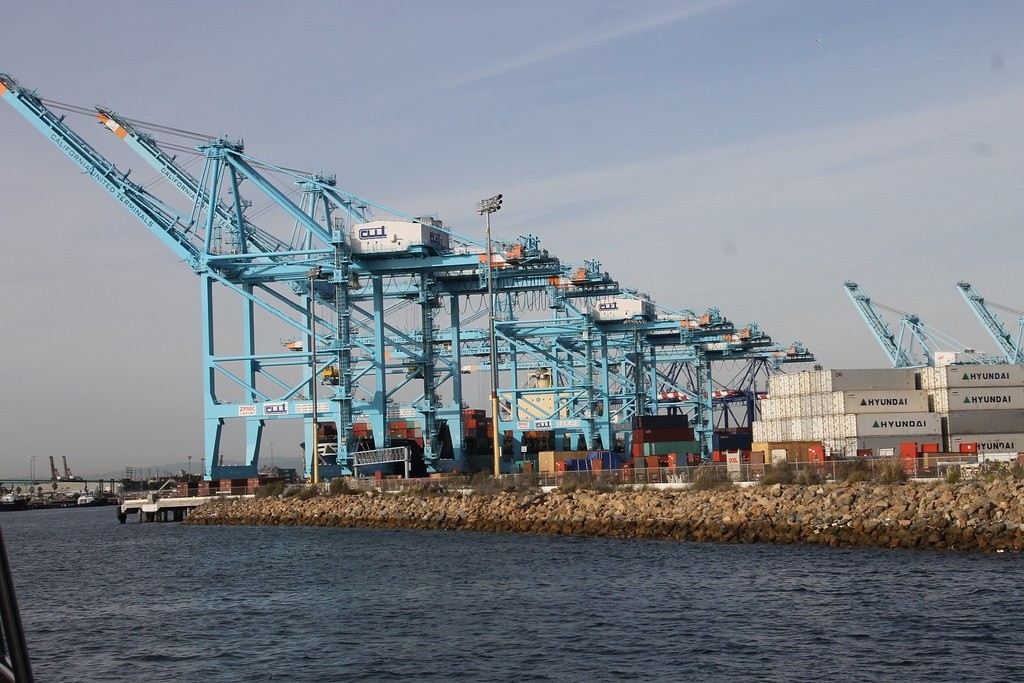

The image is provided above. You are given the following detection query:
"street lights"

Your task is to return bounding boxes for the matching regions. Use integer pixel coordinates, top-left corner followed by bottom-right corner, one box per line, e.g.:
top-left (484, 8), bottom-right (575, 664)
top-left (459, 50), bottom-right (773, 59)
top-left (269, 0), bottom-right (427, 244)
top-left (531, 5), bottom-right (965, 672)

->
top-left (304, 261), bottom-right (326, 484)
top-left (187, 455), bottom-right (192, 482)
top-left (476, 191), bottom-right (503, 478)
top-left (200, 457), bottom-right (204, 480)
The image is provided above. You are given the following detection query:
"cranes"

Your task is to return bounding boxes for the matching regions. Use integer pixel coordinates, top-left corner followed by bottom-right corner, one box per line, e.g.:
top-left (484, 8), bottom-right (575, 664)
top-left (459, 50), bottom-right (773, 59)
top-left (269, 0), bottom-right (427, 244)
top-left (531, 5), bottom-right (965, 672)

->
top-left (0, 73), bottom-right (1024, 482)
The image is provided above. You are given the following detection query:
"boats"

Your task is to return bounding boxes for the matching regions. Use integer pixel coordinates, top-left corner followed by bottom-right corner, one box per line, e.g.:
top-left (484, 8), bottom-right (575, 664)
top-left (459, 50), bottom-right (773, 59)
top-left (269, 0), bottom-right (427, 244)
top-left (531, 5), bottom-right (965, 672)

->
top-left (0, 477), bottom-right (124, 511)
